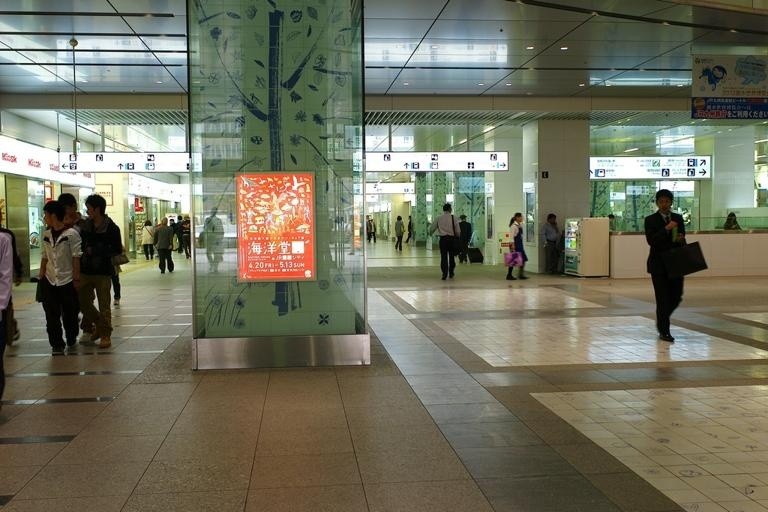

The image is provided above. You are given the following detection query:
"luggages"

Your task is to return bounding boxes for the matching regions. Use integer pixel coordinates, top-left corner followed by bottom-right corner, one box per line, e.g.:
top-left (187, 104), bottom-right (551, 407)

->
top-left (468, 248), bottom-right (483, 264)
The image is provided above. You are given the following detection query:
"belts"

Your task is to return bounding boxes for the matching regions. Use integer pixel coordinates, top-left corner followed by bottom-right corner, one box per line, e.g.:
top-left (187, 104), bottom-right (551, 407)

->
top-left (547, 239), bottom-right (556, 243)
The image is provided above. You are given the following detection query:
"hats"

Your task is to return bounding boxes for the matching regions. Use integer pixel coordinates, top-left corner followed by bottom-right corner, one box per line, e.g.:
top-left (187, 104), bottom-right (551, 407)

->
top-left (460, 215), bottom-right (467, 219)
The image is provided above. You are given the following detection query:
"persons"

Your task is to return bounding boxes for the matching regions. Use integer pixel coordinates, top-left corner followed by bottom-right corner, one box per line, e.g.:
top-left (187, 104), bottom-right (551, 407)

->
top-left (142, 214), bottom-right (192, 274)
top-left (35, 192), bottom-right (124, 355)
top-left (605, 214), bottom-right (617, 230)
top-left (202, 205), bottom-right (227, 275)
top-left (1, 227), bottom-right (24, 399)
top-left (724, 211), bottom-right (741, 231)
top-left (642, 187), bottom-right (689, 343)
top-left (364, 201), bottom-right (565, 280)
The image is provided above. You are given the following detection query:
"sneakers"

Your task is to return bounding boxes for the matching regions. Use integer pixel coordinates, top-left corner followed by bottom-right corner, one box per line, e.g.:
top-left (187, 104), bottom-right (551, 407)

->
top-left (67, 335), bottom-right (77, 345)
top-left (99, 336), bottom-right (111, 348)
top-left (52, 343), bottom-right (66, 354)
top-left (79, 332), bottom-right (97, 345)
top-left (7, 330), bottom-right (21, 346)
top-left (89, 324), bottom-right (113, 341)
top-left (114, 299), bottom-right (119, 305)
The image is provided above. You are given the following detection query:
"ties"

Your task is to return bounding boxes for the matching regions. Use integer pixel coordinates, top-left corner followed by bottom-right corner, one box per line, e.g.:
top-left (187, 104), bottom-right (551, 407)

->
top-left (665, 216), bottom-right (673, 243)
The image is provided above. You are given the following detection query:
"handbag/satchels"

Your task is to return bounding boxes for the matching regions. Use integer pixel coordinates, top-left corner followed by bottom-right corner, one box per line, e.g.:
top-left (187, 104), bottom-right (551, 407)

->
top-left (669, 241), bottom-right (708, 277)
top-left (451, 236), bottom-right (460, 256)
top-left (504, 252), bottom-right (523, 266)
top-left (401, 225), bottom-right (406, 232)
top-left (115, 254), bottom-right (129, 264)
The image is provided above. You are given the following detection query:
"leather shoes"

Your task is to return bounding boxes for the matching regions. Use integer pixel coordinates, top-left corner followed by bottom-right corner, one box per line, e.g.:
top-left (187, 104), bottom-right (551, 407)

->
top-left (519, 274), bottom-right (529, 279)
top-left (449, 273), bottom-right (454, 278)
top-left (441, 275), bottom-right (447, 280)
top-left (659, 333), bottom-right (675, 341)
top-left (506, 274), bottom-right (517, 280)
